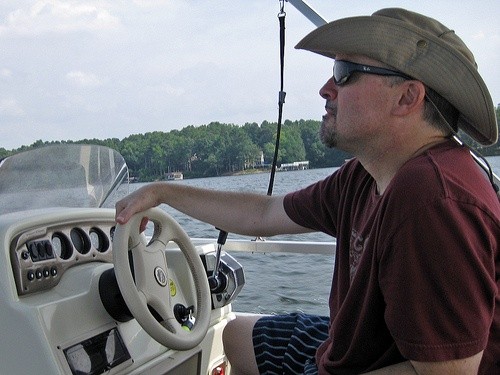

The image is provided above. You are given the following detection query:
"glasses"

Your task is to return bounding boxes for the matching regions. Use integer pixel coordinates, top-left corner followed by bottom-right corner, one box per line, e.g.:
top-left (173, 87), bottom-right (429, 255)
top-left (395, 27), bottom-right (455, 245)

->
top-left (325, 56), bottom-right (413, 87)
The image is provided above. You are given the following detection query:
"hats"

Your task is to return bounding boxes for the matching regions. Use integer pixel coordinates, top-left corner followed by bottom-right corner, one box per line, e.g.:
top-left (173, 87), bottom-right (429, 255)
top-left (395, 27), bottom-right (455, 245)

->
top-left (294, 4), bottom-right (499, 146)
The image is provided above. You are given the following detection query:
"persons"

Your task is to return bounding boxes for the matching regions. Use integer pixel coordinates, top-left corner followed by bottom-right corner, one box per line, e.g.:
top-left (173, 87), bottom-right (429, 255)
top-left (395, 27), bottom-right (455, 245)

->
top-left (114, 7), bottom-right (500, 375)
